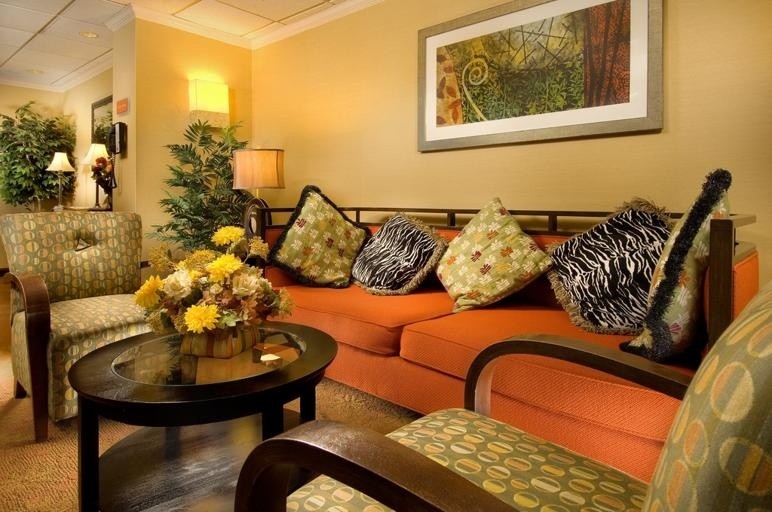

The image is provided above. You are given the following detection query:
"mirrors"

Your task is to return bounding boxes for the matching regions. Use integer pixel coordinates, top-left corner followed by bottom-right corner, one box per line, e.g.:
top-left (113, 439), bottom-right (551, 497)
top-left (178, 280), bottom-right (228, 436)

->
top-left (91, 94), bottom-right (112, 143)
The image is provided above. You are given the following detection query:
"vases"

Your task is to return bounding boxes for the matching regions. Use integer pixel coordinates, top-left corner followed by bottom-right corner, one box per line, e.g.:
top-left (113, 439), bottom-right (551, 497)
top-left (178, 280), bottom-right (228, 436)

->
top-left (179, 328), bottom-right (261, 359)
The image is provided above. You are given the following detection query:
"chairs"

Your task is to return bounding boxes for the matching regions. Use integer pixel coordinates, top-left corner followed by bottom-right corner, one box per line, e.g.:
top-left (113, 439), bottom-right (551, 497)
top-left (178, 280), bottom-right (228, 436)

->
top-left (234, 278), bottom-right (771, 512)
top-left (1, 212), bottom-right (155, 441)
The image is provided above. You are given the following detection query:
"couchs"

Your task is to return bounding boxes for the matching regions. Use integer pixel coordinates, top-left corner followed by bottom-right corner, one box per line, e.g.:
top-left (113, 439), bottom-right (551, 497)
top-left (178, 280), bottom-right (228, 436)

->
top-left (264, 208), bottom-right (757, 485)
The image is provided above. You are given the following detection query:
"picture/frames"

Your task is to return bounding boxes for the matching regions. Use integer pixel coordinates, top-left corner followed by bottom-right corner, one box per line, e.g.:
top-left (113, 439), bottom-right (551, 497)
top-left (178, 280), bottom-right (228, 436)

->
top-left (417, 0), bottom-right (662, 152)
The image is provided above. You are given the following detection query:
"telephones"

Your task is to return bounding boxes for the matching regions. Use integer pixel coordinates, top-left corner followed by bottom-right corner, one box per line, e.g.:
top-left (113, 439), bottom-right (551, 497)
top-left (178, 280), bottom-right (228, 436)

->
top-left (106, 122), bottom-right (126, 154)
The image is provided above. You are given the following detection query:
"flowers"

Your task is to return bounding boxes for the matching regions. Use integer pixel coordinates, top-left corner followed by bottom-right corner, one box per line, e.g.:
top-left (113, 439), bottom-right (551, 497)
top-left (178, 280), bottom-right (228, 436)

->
top-left (134, 227), bottom-right (294, 335)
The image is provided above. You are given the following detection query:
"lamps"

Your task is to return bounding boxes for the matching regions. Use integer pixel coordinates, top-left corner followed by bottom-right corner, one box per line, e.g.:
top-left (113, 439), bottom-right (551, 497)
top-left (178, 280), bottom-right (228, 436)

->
top-left (231, 149), bottom-right (286, 199)
top-left (188, 79), bottom-right (230, 130)
top-left (46, 152), bottom-right (75, 212)
top-left (79, 144), bottom-right (112, 212)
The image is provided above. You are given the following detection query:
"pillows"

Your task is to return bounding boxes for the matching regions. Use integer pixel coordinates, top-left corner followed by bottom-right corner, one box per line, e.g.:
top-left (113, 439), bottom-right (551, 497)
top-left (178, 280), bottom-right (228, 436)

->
top-left (543, 196), bottom-right (677, 334)
top-left (432, 197), bottom-right (555, 313)
top-left (269, 184), bottom-right (372, 289)
top-left (351, 209), bottom-right (449, 296)
top-left (618, 170), bottom-right (733, 362)
